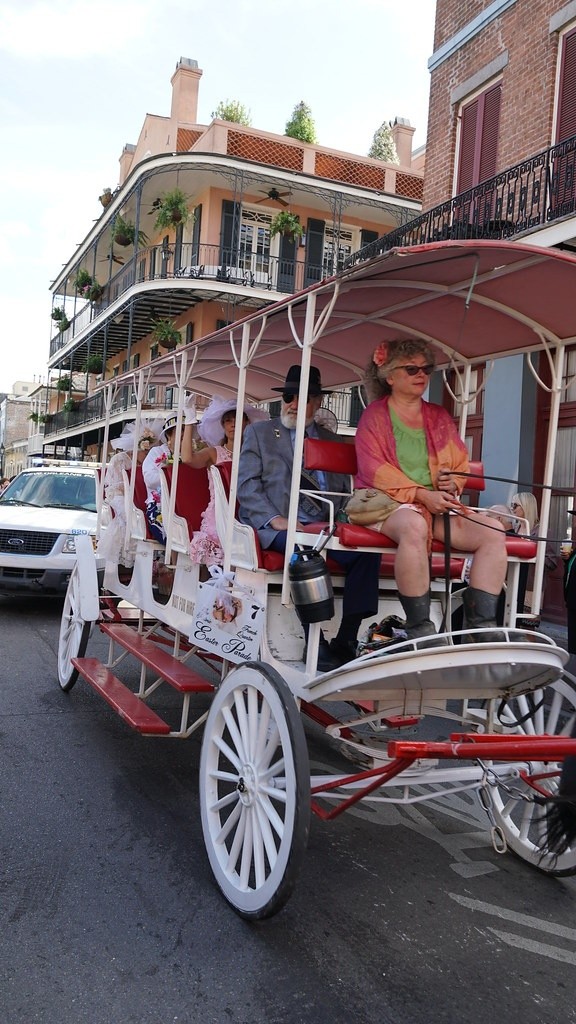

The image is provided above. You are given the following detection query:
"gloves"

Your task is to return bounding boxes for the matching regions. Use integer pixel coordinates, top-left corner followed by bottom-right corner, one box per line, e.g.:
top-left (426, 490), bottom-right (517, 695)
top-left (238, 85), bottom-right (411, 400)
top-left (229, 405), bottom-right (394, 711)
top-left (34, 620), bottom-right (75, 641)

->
top-left (183, 393), bottom-right (198, 424)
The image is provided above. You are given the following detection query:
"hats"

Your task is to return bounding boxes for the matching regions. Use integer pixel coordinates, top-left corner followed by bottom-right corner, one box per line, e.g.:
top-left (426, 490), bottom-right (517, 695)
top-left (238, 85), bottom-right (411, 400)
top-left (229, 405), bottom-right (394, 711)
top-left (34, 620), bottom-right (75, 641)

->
top-left (197, 393), bottom-right (270, 447)
top-left (270, 365), bottom-right (334, 395)
top-left (159, 412), bottom-right (188, 443)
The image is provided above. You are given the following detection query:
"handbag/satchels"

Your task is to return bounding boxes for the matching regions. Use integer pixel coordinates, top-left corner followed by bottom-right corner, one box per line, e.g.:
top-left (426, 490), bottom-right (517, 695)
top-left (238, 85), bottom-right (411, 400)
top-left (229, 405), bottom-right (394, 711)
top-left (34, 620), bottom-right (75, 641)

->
top-left (345, 490), bottom-right (404, 525)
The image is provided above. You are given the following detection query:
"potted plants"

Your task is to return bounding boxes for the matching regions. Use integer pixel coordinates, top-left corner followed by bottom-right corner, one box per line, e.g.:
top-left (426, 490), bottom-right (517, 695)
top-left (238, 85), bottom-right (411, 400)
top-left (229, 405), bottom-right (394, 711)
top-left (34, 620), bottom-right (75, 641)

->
top-left (72, 268), bottom-right (98, 296)
top-left (25, 410), bottom-right (38, 423)
top-left (97, 187), bottom-right (113, 206)
top-left (55, 374), bottom-right (77, 393)
top-left (145, 317), bottom-right (182, 350)
top-left (38, 413), bottom-right (53, 423)
top-left (51, 307), bottom-right (70, 332)
top-left (81, 279), bottom-right (103, 300)
top-left (268, 210), bottom-right (304, 244)
top-left (105, 212), bottom-right (151, 250)
top-left (79, 354), bottom-right (110, 374)
top-left (149, 188), bottom-right (199, 236)
top-left (62, 399), bottom-right (80, 422)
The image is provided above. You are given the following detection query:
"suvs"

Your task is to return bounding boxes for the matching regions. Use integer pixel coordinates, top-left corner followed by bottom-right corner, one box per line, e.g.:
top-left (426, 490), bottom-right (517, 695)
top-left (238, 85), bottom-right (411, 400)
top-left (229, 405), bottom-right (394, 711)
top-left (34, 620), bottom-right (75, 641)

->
top-left (0, 459), bottom-right (110, 608)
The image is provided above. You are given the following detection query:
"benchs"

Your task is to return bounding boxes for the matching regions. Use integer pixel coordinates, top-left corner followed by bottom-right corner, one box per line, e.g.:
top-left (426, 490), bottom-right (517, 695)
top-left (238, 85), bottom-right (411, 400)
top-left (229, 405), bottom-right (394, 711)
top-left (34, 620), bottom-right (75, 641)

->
top-left (295, 438), bottom-right (538, 563)
top-left (159, 463), bottom-right (210, 556)
top-left (210, 461), bottom-right (468, 591)
top-left (122, 466), bottom-right (161, 544)
top-left (94, 468), bottom-right (116, 529)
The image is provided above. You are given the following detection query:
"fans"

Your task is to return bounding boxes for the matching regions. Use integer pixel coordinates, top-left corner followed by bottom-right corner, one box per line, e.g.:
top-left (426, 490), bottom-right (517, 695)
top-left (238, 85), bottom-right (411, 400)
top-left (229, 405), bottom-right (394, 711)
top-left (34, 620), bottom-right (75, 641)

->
top-left (98, 252), bottom-right (125, 265)
top-left (254, 188), bottom-right (292, 208)
top-left (140, 198), bottom-right (164, 215)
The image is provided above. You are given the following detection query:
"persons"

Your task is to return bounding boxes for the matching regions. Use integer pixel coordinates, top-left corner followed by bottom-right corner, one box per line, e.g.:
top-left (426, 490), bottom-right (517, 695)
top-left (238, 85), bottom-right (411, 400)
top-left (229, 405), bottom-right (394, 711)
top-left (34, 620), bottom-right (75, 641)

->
top-left (181, 393), bottom-right (270, 592)
top-left (237, 365), bottom-right (383, 673)
top-left (0, 476), bottom-right (23, 502)
top-left (560, 510), bottom-right (576, 657)
top-left (464, 493), bottom-right (559, 642)
top-left (353, 339), bottom-right (507, 653)
top-left (28, 477), bottom-right (61, 506)
top-left (142, 412), bottom-right (201, 577)
top-left (104, 414), bottom-right (167, 568)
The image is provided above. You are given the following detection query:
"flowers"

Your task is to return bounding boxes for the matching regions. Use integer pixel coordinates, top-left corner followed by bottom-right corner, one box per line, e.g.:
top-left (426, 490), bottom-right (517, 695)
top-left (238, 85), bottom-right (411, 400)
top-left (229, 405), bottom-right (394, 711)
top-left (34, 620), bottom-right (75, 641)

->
top-left (372, 342), bottom-right (388, 366)
top-left (151, 489), bottom-right (162, 504)
top-left (156, 454), bottom-right (173, 470)
top-left (138, 427), bottom-right (156, 451)
top-left (368, 120), bottom-right (401, 166)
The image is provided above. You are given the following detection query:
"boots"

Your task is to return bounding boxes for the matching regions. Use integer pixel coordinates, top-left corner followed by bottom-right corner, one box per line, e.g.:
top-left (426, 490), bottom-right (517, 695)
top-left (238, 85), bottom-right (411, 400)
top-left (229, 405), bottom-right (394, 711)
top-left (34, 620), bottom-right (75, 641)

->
top-left (398, 591), bottom-right (447, 649)
top-left (463, 586), bottom-right (528, 644)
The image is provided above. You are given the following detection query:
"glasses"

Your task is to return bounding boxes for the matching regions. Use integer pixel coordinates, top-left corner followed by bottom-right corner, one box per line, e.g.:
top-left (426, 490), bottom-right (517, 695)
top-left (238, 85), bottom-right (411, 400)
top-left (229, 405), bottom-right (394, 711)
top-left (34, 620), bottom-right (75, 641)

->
top-left (281, 393), bottom-right (319, 404)
top-left (389, 364), bottom-right (435, 375)
top-left (510, 502), bottom-right (523, 509)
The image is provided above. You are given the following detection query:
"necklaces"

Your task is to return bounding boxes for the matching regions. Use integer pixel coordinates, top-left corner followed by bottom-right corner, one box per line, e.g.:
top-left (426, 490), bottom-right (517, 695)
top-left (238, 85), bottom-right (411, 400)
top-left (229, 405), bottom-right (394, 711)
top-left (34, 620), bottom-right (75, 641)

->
top-left (131, 453), bottom-right (143, 464)
top-left (224, 444), bottom-right (233, 453)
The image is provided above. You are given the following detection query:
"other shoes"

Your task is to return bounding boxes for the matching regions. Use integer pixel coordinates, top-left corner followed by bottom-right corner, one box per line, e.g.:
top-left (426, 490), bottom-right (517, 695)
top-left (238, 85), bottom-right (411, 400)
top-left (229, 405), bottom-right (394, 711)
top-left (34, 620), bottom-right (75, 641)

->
top-left (330, 638), bottom-right (359, 663)
top-left (300, 640), bottom-right (343, 672)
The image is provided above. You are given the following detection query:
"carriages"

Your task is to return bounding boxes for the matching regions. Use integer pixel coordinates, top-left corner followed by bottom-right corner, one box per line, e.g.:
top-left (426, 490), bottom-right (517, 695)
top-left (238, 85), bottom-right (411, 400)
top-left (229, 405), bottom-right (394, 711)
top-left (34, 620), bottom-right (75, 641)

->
top-left (57, 238), bottom-right (576, 920)
